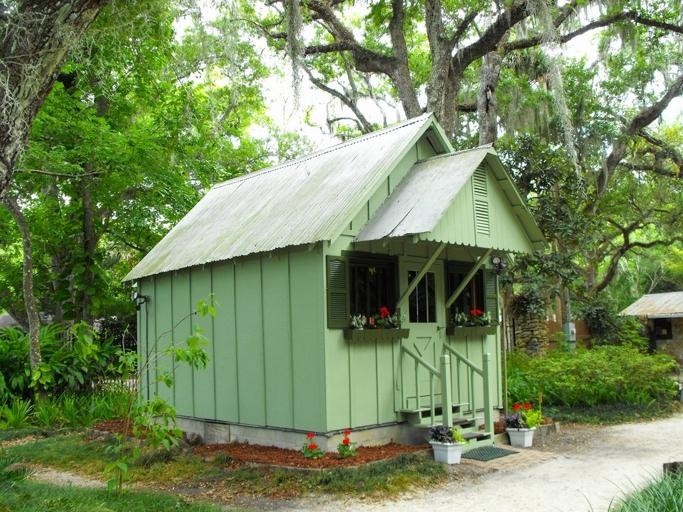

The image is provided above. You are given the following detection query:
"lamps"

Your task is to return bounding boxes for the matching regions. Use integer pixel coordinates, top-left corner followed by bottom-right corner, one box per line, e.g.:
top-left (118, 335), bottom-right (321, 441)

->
top-left (491, 255), bottom-right (508, 270)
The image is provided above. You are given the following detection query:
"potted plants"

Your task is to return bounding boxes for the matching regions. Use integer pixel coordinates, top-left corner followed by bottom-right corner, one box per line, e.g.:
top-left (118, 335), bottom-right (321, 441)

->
top-left (502, 409), bottom-right (540, 448)
top-left (428, 426), bottom-right (466, 466)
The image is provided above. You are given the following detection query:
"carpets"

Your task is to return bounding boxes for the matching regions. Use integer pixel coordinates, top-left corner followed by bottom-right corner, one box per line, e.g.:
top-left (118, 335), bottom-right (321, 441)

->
top-left (461, 446), bottom-right (519, 461)
